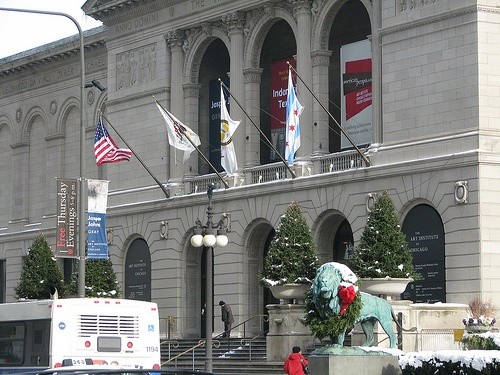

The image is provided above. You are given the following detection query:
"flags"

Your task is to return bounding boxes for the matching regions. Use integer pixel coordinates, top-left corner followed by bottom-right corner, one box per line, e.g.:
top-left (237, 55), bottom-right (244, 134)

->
top-left (94, 119), bottom-right (133, 165)
top-left (153, 100), bottom-right (201, 159)
top-left (219, 86), bottom-right (242, 175)
top-left (282, 69), bottom-right (302, 166)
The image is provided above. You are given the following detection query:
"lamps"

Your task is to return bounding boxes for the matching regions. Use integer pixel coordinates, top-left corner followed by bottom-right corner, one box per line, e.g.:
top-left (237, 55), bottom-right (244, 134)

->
top-left (276, 172), bottom-right (279, 180)
top-left (195, 186), bottom-right (198, 193)
top-left (258, 175), bottom-right (262, 183)
top-left (329, 163), bottom-right (333, 172)
top-left (307, 167), bottom-right (311, 176)
top-left (240, 179), bottom-right (244, 185)
top-left (349, 159), bottom-right (354, 168)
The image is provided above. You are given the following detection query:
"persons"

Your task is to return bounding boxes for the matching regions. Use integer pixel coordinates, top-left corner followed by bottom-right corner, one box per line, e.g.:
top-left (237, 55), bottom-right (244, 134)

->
top-left (284, 346), bottom-right (308, 375)
top-left (219, 301), bottom-right (234, 338)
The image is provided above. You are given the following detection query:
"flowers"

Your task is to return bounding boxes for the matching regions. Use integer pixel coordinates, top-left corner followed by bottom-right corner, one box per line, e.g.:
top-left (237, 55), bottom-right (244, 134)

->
top-left (462, 297), bottom-right (497, 326)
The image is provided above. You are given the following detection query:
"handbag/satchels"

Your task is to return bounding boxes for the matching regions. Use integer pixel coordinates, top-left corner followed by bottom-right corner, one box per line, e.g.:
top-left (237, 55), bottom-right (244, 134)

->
top-left (301, 355), bottom-right (310, 374)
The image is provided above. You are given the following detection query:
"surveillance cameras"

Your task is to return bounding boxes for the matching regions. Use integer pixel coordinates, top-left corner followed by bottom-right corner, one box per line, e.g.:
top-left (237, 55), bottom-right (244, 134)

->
top-left (91, 79), bottom-right (105, 92)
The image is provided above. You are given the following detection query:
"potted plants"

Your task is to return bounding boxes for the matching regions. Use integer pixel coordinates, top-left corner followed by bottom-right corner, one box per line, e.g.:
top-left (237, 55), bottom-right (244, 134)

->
top-left (15, 233), bottom-right (70, 302)
top-left (258, 201), bottom-right (323, 305)
top-left (461, 335), bottom-right (498, 350)
top-left (354, 191), bottom-right (420, 300)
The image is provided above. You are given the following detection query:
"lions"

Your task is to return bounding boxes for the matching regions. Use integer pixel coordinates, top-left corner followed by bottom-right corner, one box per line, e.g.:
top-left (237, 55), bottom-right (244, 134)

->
top-left (311, 261), bottom-right (418, 349)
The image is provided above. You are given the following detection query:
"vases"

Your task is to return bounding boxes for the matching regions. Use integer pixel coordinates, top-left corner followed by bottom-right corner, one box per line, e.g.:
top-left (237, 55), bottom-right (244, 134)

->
top-left (466, 326), bottom-right (498, 334)
